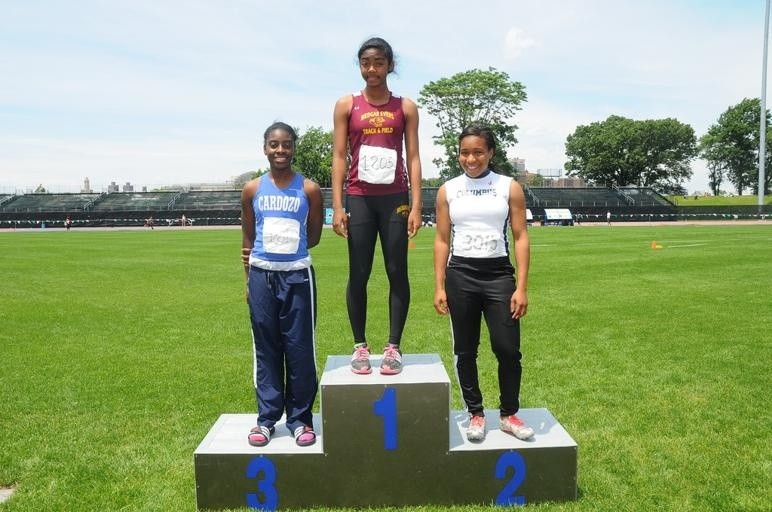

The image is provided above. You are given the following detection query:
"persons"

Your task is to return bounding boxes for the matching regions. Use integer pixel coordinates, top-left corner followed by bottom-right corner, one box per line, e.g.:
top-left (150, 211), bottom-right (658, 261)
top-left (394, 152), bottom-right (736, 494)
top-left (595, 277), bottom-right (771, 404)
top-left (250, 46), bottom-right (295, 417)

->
top-left (433, 125), bottom-right (534, 441)
top-left (65, 215), bottom-right (72, 231)
top-left (240, 122), bottom-right (323, 446)
top-left (606, 210), bottom-right (612, 226)
top-left (181, 213), bottom-right (187, 229)
top-left (332, 37), bottom-right (422, 374)
top-left (144, 215), bottom-right (155, 230)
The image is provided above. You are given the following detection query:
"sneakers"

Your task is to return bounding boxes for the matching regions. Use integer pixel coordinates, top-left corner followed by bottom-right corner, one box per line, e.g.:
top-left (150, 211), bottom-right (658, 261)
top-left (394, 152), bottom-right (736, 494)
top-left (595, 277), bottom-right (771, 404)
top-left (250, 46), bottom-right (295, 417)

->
top-left (500, 415), bottom-right (533, 440)
top-left (351, 343), bottom-right (371, 374)
top-left (467, 415), bottom-right (484, 440)
top-left (381, 343), bottom-right (402, 374)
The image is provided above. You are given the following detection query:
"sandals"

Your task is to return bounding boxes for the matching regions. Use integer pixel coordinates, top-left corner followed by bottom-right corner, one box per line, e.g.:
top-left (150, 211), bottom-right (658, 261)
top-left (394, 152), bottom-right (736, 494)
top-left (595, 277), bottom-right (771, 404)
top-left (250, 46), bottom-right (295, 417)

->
top-left (248, 426), bottom-right (275, 446)
top-left (294, 426), bottom-right (315, 445)
